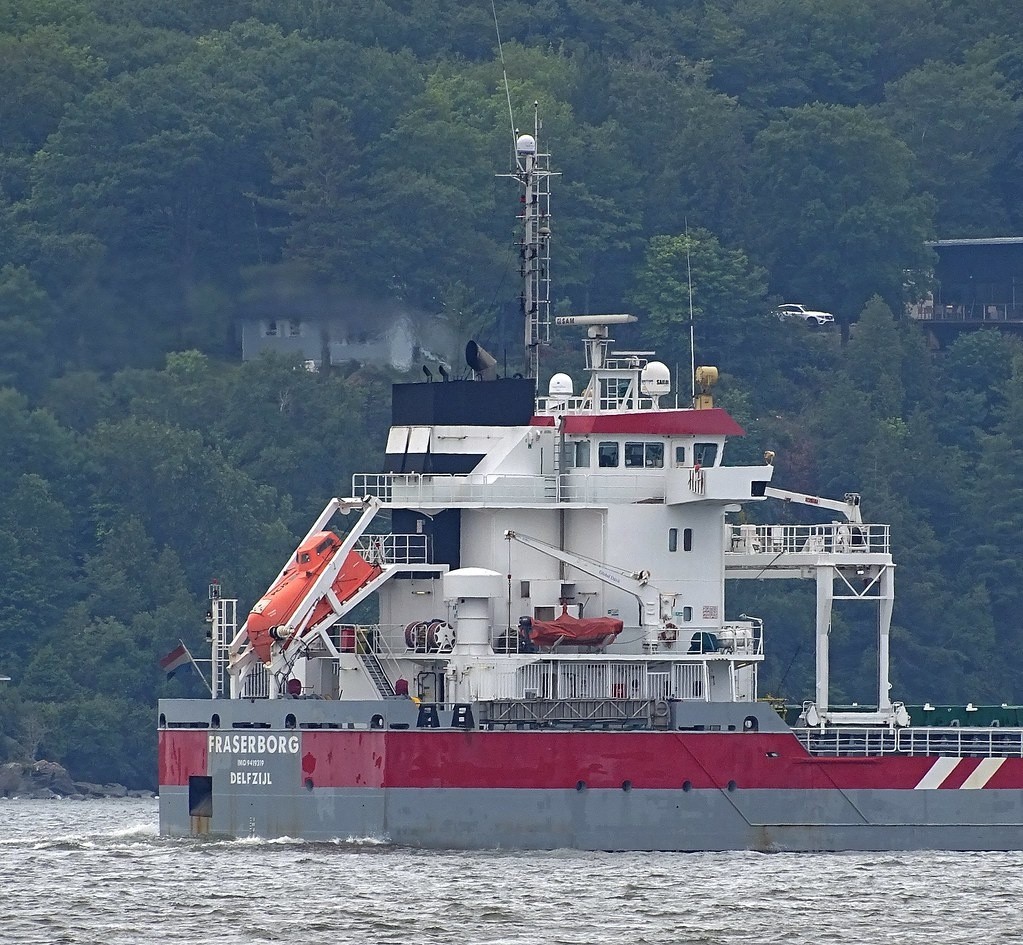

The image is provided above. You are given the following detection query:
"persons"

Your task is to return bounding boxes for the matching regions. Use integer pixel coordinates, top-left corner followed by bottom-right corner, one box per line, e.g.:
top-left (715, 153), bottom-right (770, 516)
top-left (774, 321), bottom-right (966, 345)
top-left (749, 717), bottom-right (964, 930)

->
top-left (929, 718), bottom-right (1020, 758)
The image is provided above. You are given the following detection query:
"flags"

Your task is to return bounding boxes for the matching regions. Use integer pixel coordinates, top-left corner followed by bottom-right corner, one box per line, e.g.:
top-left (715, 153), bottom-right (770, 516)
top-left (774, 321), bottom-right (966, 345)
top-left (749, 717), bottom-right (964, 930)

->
top-left (159, 643), bottom-right (193, 680)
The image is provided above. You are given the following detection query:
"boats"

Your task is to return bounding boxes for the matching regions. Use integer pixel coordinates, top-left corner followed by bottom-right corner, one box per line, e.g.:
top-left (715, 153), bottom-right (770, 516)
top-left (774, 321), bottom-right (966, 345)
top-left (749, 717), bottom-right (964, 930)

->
top-left (155, 0), bottom-right (1023, 851)
top-left (520, 601), bottom-right (623, 645)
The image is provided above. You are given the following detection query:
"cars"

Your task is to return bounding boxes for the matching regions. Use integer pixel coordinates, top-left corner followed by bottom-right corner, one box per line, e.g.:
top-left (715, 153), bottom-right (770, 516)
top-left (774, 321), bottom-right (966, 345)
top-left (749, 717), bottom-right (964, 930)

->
top-left (769, 303), bottom-right (835, 328)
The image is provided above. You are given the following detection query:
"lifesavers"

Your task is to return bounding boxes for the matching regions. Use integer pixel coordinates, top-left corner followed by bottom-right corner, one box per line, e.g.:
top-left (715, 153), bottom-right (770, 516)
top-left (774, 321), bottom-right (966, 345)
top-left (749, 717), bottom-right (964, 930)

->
top-left (662, 623), bottom-right (679, 642)
